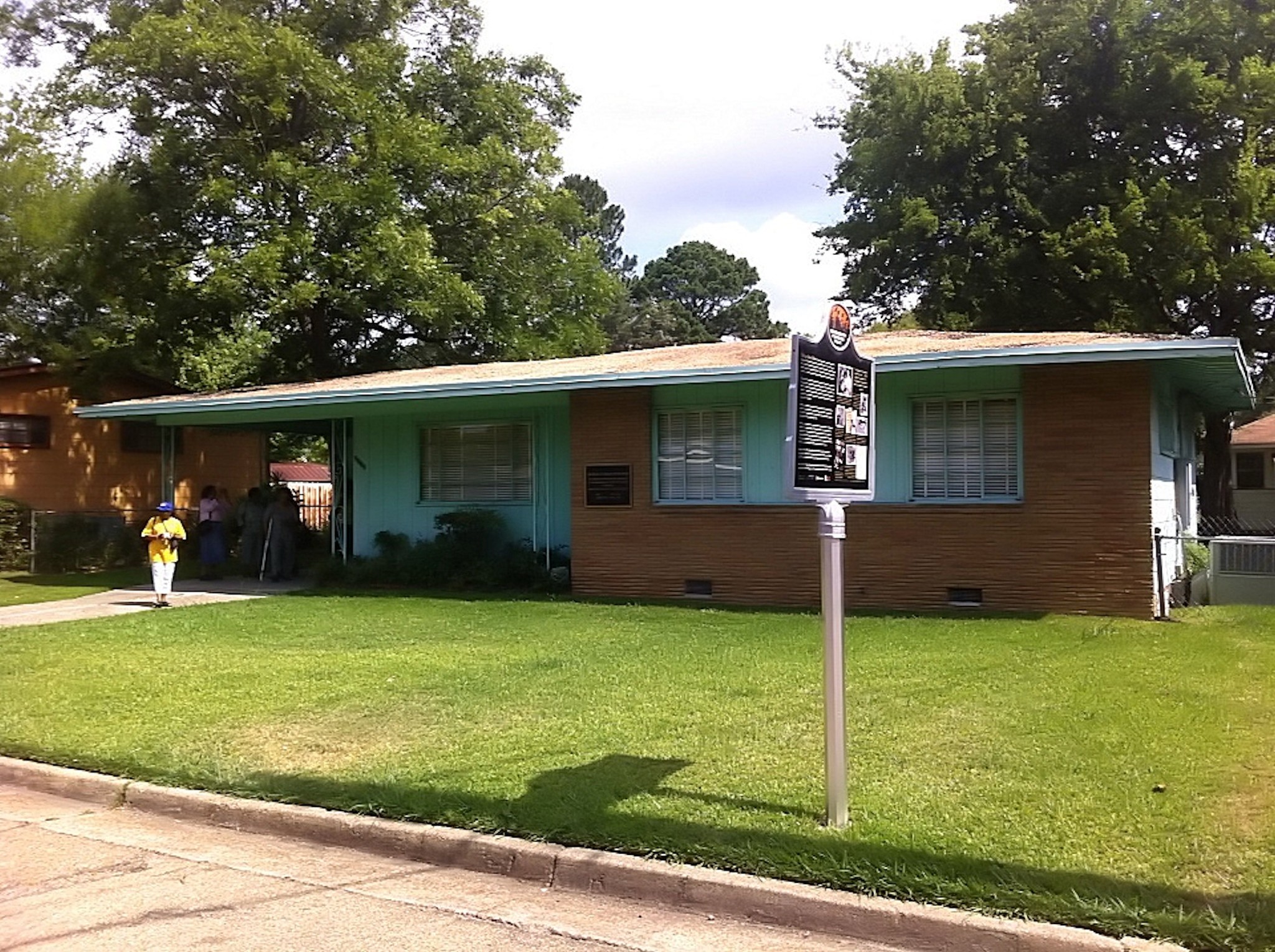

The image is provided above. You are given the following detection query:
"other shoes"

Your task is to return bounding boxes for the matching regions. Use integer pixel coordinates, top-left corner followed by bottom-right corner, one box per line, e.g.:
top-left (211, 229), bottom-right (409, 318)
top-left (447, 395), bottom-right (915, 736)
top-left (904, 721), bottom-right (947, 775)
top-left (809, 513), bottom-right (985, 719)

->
top-left (200, 574), bottom-right (223, 580)
top-left (154, 600), bottom-right (172, 609)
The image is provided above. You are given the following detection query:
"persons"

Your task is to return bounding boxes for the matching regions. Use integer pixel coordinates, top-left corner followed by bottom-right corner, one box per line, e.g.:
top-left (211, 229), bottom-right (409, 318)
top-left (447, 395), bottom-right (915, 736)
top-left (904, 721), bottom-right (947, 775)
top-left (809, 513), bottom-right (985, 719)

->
top-left (141, 502), bottom-right (186, 606)
top-left (236, 487), bottom-right (264, 576)
top-left (199, 485), bottom-right (231, 580)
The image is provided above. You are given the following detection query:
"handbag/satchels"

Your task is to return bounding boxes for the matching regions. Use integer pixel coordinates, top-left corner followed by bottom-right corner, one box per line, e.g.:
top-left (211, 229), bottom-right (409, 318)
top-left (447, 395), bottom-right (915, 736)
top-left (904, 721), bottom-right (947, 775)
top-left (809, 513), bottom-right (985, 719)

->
top-left (170, 540), bottom-right (179, 552)
top-left (193, 519), bottom-right (213, 540)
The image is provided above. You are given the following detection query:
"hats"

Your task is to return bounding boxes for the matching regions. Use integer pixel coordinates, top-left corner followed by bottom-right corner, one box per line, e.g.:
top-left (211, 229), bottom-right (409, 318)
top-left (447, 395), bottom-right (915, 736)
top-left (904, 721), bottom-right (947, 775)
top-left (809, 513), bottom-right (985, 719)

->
top-left (155, 501), bottom-right (173, 512)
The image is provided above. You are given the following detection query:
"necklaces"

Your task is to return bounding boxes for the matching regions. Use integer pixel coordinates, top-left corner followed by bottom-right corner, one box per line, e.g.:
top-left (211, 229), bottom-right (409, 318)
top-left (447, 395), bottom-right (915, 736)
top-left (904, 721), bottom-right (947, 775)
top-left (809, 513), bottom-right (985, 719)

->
top-left (265, 489), bottom-right (300, 581)
